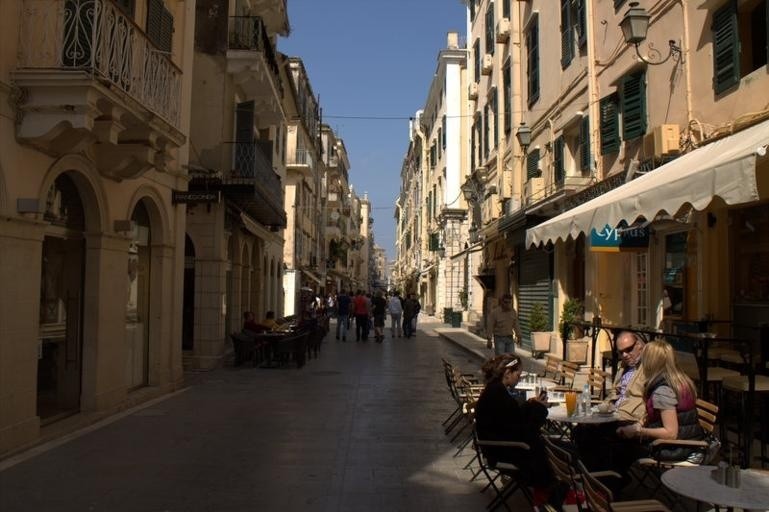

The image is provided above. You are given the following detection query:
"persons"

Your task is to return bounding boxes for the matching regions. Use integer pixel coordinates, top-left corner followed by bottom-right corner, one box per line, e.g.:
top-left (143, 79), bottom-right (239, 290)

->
top-left (486, 292), bottom-right (523, 356)
top-left (243, 287), bottom-right (422, 364)
top-left (583, 337), bottom-right (702, 501)
top-left (474, 352), bottom-right (586, 511)
top-left (569, 328), bottom-right (646, 490)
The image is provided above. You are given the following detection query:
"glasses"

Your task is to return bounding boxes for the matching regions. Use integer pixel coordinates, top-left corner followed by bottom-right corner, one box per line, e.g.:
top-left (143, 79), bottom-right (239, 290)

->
top-left (617, 340), bottom-right (637, 354)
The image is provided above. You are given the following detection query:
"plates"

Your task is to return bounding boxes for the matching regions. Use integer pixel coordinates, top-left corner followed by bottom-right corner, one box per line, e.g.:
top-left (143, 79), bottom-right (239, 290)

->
top-left (594, 410), bottom-right (616, 417)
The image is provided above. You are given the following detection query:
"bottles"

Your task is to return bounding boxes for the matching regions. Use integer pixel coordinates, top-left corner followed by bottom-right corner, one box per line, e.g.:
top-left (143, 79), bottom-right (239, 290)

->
top-left (264, 324), bottom-right (291, 335)
top-left (717, 463), bottom-right (741, 488)
top-left (565, 383), bottom-right (591, 418)
top-left (519, 371), bottom-right (537, 383)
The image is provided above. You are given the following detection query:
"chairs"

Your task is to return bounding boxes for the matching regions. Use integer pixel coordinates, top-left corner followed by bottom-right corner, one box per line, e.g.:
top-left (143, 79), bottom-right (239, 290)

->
top-left (230, 310), bottom-right (332, 369)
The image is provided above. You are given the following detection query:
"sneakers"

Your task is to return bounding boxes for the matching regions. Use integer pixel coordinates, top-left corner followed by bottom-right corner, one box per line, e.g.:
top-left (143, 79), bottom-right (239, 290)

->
top-left (336, 335), bottom-right (416, 343)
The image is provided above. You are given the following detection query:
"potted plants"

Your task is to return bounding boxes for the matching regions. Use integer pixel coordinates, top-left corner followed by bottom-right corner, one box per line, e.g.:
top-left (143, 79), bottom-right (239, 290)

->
top-left (528, 300), bottom-right (553, 352)
top-left (559, 297), bottom-right (588, 363)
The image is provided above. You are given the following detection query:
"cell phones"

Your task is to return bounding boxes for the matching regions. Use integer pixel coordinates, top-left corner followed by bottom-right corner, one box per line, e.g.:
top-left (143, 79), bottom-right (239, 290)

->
top-left (540, 391), bottom-right (546, 400)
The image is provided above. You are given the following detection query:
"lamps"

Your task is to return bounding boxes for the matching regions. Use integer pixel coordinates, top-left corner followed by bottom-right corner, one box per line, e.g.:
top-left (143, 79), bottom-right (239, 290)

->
top-left (114, 219), bottom-right (134, 232)
top-left (16, 197), bottom-right (45, 214)
top-left (516, 122), bottom-right (553, 161)
top-left (619, 2), bottom-right (685, 68)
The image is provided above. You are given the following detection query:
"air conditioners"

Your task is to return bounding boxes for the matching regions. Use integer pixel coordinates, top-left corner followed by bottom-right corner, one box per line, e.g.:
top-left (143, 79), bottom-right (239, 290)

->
top-left (642, 124), bottom-right (681, 159)
top-left (467, 18), bottom-right (511, 100)
